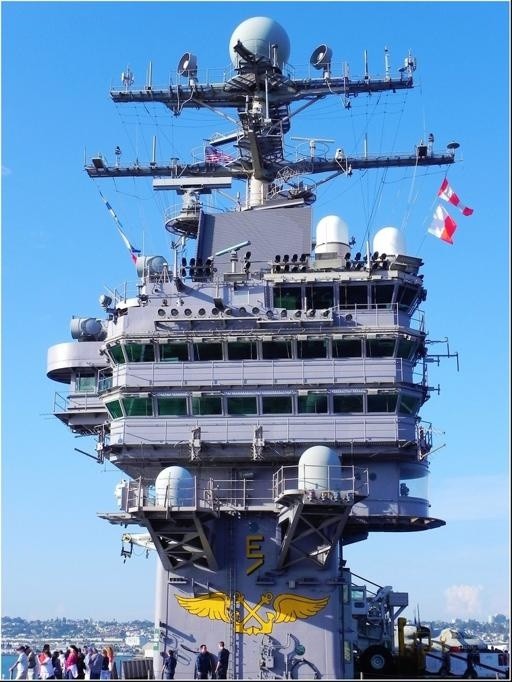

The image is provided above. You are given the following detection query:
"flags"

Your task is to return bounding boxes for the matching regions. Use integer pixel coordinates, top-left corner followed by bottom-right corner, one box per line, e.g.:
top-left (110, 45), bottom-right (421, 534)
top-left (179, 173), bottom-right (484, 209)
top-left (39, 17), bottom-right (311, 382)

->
top-left (436, 177), bottom-right (473, 216)
top-left (427, 204), bottom-right (457, 245)
top-left (204, 145), bottom-right (235, 164)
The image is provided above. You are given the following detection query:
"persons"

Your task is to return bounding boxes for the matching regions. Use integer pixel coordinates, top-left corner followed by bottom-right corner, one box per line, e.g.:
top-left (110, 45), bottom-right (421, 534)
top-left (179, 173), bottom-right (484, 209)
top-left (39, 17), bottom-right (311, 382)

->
top-left (215, 641), bottom-right (229, 679)
top-left (8, 644), bottom-right (114, 680)
top-left (161, 650), bottom-right (177, 679)
top-left (196, 645), bottom-right (213, 679)
